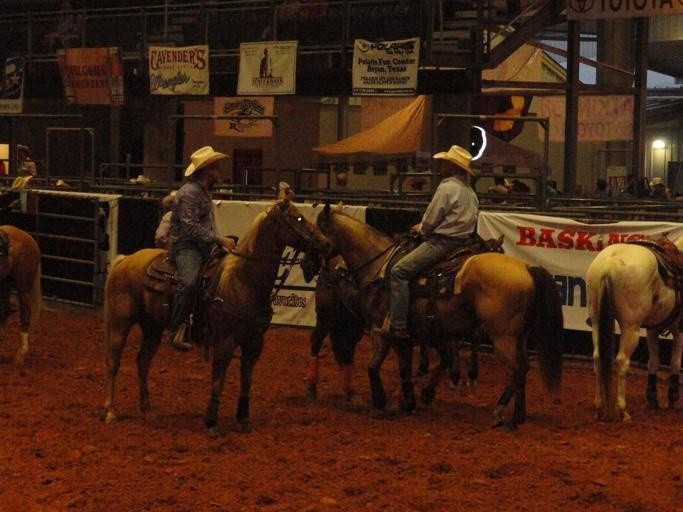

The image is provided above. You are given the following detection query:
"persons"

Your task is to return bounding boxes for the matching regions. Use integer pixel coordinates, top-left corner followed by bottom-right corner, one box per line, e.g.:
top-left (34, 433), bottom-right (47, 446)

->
top-left (304, 257), bottom-right (363, 403)
top-left (15, 142), bottom-right (38, 178)
top-left (54, 171), bottom-right (233, 200)
top-left (371, 144), bottom-right (480, 340)
top-left (259, 47), bottom-right (273, 77)
top-left (167, 143), bottom-right (235, 353)
top-left (406, 172), bottom-right (683, 220)
top-left (154, 188), bottom-right (178, 249)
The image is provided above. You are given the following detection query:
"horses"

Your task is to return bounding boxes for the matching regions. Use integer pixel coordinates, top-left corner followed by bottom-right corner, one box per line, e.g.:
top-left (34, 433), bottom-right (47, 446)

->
top-left (586, 229), bottom-right (683, 425)
top-left (299, 200), bottom-right (564, 434)
top-left (0, 225), bottom-right (41, 364)
top-left (103, 187), bottom-right (333, 438)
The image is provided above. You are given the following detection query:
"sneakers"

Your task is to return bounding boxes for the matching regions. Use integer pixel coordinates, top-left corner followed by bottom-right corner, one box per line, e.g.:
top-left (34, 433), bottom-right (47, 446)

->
top-left (167, 338), bottom-right (191, 352)
top-left (375, 326), bottom-right (409, 339)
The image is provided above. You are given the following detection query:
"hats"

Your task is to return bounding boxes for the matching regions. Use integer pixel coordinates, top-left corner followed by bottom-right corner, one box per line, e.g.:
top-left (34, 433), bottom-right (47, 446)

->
top-left (432, 144), bottom-right (476, 178)
top-left (184, 146), bottom-right (227, 177)
